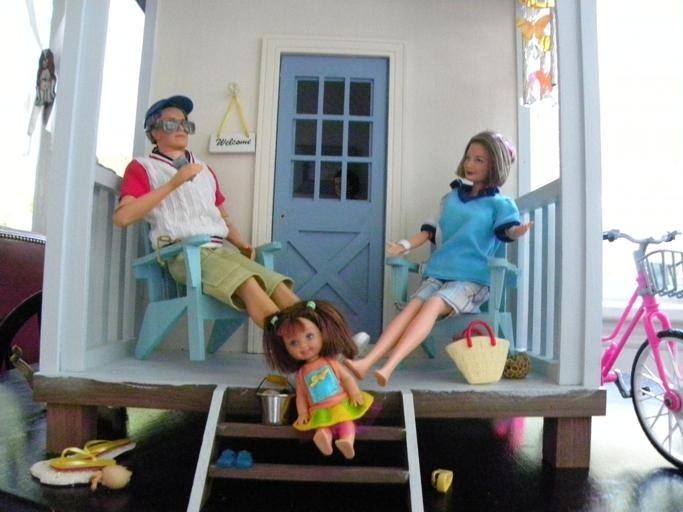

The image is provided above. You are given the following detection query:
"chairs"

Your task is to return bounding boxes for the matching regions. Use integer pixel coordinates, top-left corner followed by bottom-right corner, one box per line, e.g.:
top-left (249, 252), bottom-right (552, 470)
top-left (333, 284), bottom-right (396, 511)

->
top-left (386, 239), bottom-right (520, 371)
top-left (132, 219), bottom-right (282, 362)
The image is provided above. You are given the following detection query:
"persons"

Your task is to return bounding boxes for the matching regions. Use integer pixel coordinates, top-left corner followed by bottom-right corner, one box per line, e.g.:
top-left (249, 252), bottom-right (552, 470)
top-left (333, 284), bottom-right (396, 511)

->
top-left (112, 95), bottom-right (302, 330)
top-left (262, 299), bottom-right (374, 458)
top-left (344, 131), bottom-right (536, 387)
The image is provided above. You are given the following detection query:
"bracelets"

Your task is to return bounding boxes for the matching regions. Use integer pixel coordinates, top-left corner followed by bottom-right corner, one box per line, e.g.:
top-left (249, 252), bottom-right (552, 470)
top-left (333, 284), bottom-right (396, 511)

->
top-left (397, 239), bottom-right (410, 256)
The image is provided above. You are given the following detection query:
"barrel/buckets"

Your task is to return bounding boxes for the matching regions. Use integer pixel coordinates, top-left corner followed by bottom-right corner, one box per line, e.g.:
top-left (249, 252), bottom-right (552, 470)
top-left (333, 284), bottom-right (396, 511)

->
top-left (256, 376), bottom-right (294, 425)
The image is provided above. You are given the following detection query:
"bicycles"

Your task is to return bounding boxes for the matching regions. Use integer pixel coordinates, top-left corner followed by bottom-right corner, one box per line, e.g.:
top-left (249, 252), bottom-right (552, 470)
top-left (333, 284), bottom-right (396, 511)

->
top-left (498, 230), bottom-right (679, 459)
